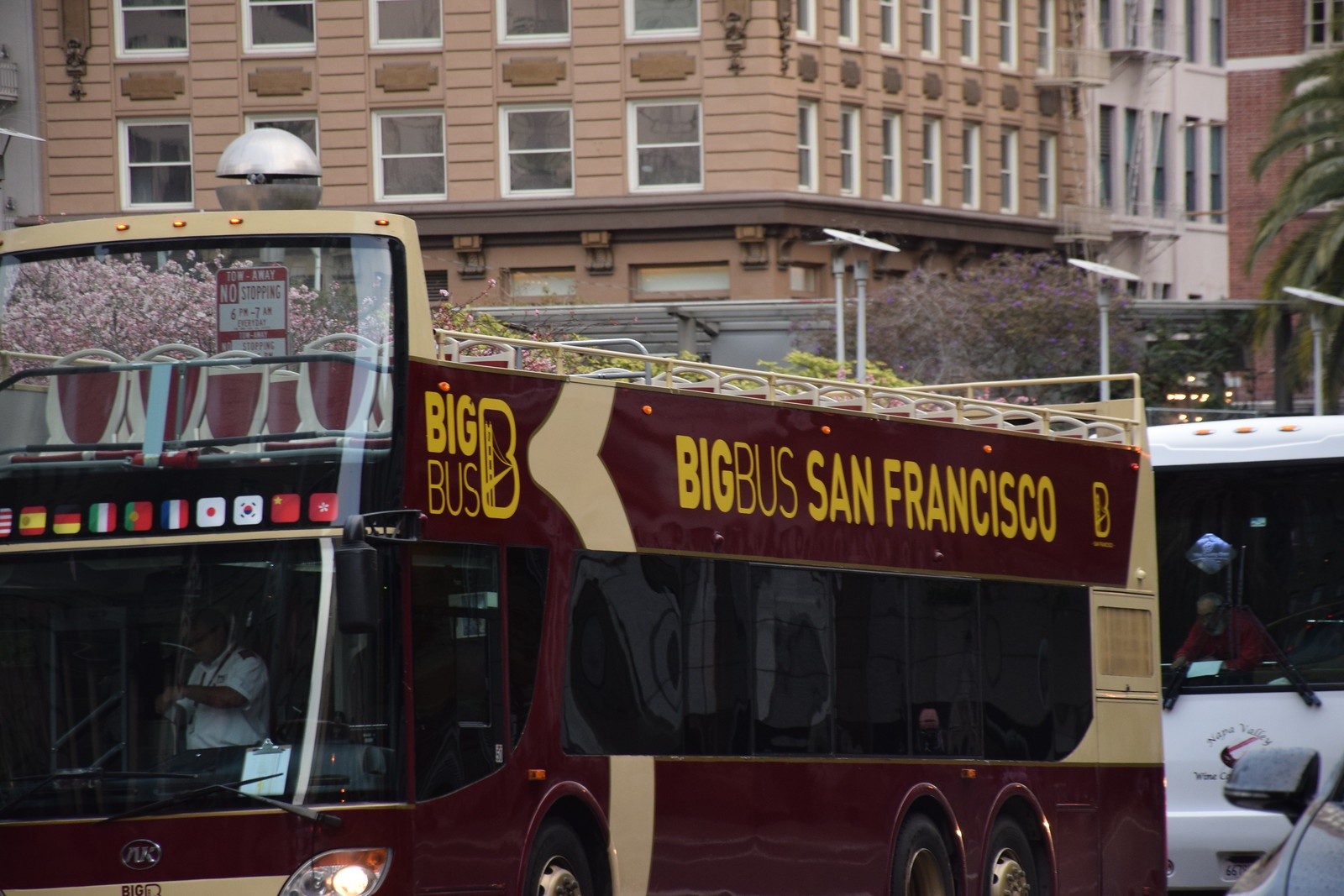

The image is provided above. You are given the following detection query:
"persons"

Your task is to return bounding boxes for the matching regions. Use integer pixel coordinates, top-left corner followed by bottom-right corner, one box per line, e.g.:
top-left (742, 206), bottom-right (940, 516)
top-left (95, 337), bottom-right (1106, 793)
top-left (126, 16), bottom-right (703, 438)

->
top-left (1172, 591), bottom-right (1266, 686)
top-left (156, 605), bottom-right (272, 755)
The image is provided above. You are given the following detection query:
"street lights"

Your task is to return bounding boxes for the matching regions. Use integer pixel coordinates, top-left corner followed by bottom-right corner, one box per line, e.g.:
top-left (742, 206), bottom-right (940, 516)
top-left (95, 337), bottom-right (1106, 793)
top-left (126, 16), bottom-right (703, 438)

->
top-left (810, 226), bottom-right (903, 395)
top-left (1283, 287), bottom-right (1344, 415)
top-left (1065, 256), bottom-right (1142, 401)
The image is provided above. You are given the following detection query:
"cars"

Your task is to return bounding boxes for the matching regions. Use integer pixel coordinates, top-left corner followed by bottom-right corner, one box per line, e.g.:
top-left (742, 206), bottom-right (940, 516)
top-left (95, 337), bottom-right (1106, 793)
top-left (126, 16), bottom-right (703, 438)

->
top-left (1219, 749), bottom-right (1343, 895)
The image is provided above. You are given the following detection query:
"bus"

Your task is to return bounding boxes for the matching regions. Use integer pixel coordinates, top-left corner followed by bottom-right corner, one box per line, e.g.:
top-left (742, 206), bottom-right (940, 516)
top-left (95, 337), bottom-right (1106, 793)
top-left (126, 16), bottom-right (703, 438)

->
top-left (0, 209), bottom-right (1171, 896)
top-left (1135, 414), bottom-right (1344, 894)
